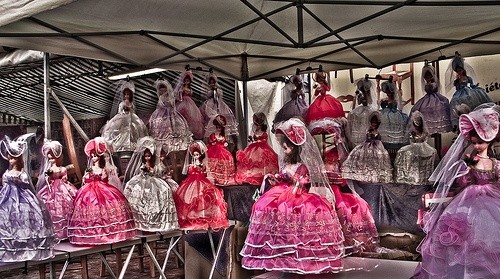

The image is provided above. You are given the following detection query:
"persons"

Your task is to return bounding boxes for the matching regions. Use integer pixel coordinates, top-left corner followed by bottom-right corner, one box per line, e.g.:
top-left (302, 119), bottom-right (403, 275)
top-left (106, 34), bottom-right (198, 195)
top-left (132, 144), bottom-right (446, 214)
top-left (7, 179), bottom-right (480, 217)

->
top-left (206, 115), bottom-right (240, 186)
top-left (405, 101), bottom-right (499, 279)
top-left (169, 69), bottom-right (206, 140)
top-left (66, 137), bottom-right (141, 246)
top-left (147, 78), bottom-right (193, 154)
top-left (303, 117), bottom-right (383, 260)
top-left (303, 65), bottom-right (348, 146)
top-left (199, 68), bottom-right (237, 148)
top-left (99, 143), bottom-right (124, 194)
top-left (100, 78), bottom-right (149, 157)
top-left (339, 110), bottom-right (411, 241)
top-left (409, 65), bottom-right (462, 160)
top-left (441, 52), bottom-right (500, 132)
top-left (392, 112), bottom-right (445, 246)
top-left (272, 68), bottom-right (310, 148)
top-left (0, 132), bottom-right (58, 265)
top-left (340, 75), bottom-right (383, 150)
top-left (153, 143), bottom-right (180, 198)
top-left (119, 136), bottom-right (182, 233)
top-left (239, 117), bottom-right (346, 279)
top-left (376, 77), bottom-right (411, 176)
top-left (172, 140), bottom-right (232, 234)
top-left (32, 139), bottom-right (80, 247)
top-left (235, 112), bottom-right (283, 185)
